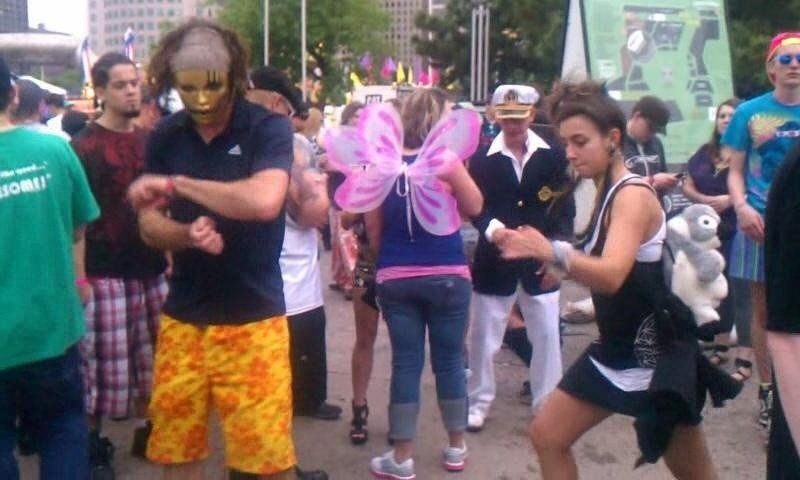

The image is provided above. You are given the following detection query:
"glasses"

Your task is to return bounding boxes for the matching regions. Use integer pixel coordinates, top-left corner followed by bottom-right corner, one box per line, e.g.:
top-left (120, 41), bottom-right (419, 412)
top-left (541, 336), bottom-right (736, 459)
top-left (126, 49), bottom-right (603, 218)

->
top-left (772, 52), bottom-right (800, 65)
top-left (273, 92), bottom-right (296, 121)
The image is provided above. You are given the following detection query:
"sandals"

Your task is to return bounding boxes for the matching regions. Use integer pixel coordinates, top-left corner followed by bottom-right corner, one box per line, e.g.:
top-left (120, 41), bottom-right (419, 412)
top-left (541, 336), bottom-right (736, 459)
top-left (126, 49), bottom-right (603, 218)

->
top-left (707, 346), bottom-right (730, 366)
top-left (726, 360), bottom-right (754, 383)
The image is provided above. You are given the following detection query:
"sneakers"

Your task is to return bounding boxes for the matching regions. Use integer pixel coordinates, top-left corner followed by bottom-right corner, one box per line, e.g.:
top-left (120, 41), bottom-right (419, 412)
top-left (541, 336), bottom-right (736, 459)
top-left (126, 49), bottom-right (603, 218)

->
top-left (85, 434), bottom-right (119, 480)
top-left (368, 451), bottom-right (417, 480)
top-left (442, 442), bottom-right (470, 473)
top-left (294, 400), bottom-right (344, 420)
top-left (753, 383), bottom-right (775, 428)
top-left (467, 410), bottom-right (486, 433)
top-left (517, 380), bottom-right (533, 406)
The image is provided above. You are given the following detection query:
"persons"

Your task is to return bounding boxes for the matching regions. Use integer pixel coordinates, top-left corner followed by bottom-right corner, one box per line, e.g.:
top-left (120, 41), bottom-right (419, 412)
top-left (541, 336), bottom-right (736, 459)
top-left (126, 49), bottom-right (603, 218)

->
top-left (8, 31), bottom-right (799, 455)
top-left (125, 23), bottom-right (297, 480)
top-left (229, 67), bottom-right (330, 480)
top-left (763, 141), bottom-right (800, 480)
top-left (365, 89), bottom-right (484, 480)
top-left (69, 52), bottom-right (174, 480)
top-left (0, 57), bottom-right (89, 480)
top-left (505, 87), bottom-right (718, 480)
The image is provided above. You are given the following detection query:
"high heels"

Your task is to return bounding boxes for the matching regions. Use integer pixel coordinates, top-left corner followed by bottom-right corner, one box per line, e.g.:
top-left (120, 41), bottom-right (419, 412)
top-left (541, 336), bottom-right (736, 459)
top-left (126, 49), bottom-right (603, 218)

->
top-left (348, 400), bottom-right (370, 445)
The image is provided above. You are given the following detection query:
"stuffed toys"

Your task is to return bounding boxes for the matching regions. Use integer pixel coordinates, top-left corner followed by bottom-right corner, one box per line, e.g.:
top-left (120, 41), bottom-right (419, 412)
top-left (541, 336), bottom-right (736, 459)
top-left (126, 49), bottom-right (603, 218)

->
top-left (667, 204), bottom-right (729, 325)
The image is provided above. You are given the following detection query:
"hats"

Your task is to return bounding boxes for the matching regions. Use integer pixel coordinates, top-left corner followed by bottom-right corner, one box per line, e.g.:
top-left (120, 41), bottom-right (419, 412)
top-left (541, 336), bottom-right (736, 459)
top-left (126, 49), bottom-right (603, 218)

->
top-left (766, 32), bottom-right (800, 62)
top-left (250, 65), bottom-right (305, 117)
top-left (642, 96), bottom-right (668, 136)
top-left (491, 83), bottom-right (540, 120)
